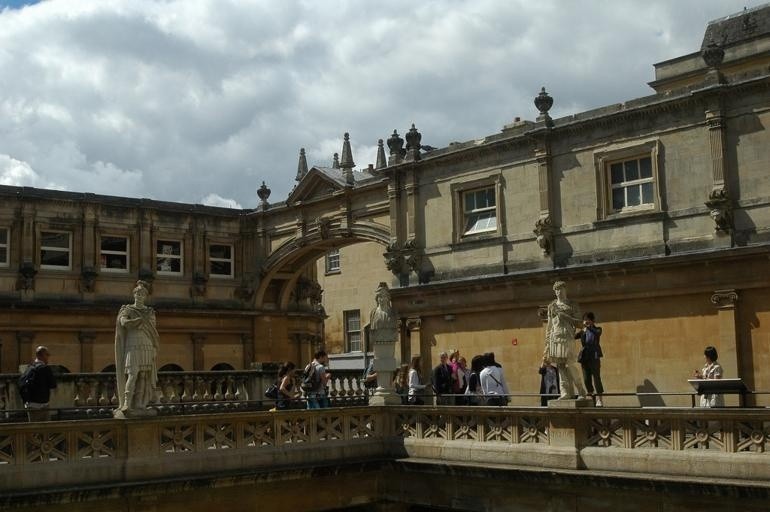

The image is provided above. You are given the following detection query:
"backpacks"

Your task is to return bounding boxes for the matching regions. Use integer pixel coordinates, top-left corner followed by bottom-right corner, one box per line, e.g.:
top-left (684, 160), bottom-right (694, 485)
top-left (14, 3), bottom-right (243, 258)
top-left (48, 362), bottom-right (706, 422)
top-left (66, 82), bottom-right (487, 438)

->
top-left (18, 366), bottom-right (38, 401)
top-left (301, 363), bottom-right (320, 391)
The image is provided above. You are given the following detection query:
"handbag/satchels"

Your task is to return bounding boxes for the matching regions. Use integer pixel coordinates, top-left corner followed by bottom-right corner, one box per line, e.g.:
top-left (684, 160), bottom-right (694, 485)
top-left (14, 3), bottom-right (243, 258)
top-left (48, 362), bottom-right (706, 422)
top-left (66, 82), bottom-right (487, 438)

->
top-left (265, 384), bottom-right (278, 398)
top-left (578, 347), bottom-right (593, 365)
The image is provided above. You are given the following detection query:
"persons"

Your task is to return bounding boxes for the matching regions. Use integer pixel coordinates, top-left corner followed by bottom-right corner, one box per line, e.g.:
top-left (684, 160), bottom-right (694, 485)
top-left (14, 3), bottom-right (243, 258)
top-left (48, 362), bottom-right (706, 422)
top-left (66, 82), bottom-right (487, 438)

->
top-left (26, 345), bottom-right (57, 422)
top-left (692, 345), bottom-right (724, 408)
top-left (544, 280), bottom-right (587, 399)
top-left (114, 281), bottom-right (160, 411)
top-left (363, 351), bottom-right (512, 405)
top-left (275, 360), bottom-right (301, 410)
top-left (574, 312), bottom-right (603, 408)
top-left (371, 282), bottom-right (397, 342)
top-left (539, 357), bottom-right (561, 406)
top-left (305, 350), bottom-right (331, 409)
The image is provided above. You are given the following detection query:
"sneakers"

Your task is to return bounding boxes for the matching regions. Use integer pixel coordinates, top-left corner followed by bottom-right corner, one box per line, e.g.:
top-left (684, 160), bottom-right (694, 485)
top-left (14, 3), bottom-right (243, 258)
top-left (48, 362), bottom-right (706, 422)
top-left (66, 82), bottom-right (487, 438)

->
top-left (596, 400), bottom-right (603, 407)
